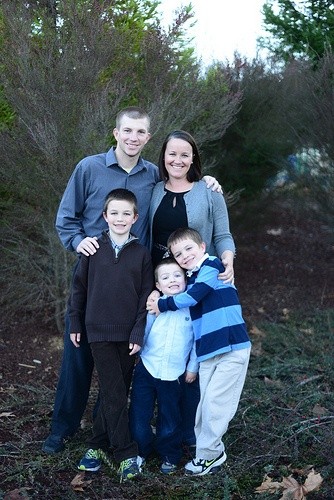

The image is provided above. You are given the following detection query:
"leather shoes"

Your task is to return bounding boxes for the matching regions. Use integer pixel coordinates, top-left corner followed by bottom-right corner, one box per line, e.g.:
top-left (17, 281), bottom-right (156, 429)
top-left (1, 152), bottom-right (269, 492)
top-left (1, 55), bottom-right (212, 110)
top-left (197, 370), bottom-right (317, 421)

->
top-left (42, 434), bottom-right (64, 453)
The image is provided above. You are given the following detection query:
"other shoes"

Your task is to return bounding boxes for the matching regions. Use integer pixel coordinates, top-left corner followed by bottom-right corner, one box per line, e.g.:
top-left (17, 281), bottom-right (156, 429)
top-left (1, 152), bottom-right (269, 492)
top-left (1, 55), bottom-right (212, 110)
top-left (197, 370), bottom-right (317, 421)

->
top-left (78, 448), bottom-right (104, 472)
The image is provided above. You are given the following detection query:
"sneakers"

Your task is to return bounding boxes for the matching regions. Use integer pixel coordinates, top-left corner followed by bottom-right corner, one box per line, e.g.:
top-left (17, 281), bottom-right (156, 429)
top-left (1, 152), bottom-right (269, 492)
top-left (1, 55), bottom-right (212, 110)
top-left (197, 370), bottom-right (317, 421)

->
top-left (184, 450), bottom-right (227, 476)
top-left (160, 456), bottom-right (181, 474)
top-left (117, 457), bottom-right (140, 484)
top-left (137, 455), bottom-right (146, 474)
top-left (187, 443), bottom-right (197, 458)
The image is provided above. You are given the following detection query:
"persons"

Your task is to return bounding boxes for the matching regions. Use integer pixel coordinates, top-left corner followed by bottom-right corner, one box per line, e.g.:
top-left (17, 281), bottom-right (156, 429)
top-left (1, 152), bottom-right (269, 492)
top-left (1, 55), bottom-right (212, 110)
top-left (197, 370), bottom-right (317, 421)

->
top-left (154, 130), bottom-right (237, 284)
top-left (146, 228), bottom-right (251, 476)
top-left (131, 258), bottom-right (199, 475)
top-left (42, 106), bottom-right (224, 453)
top-left (67, 189), bottom-right (154, 482)
